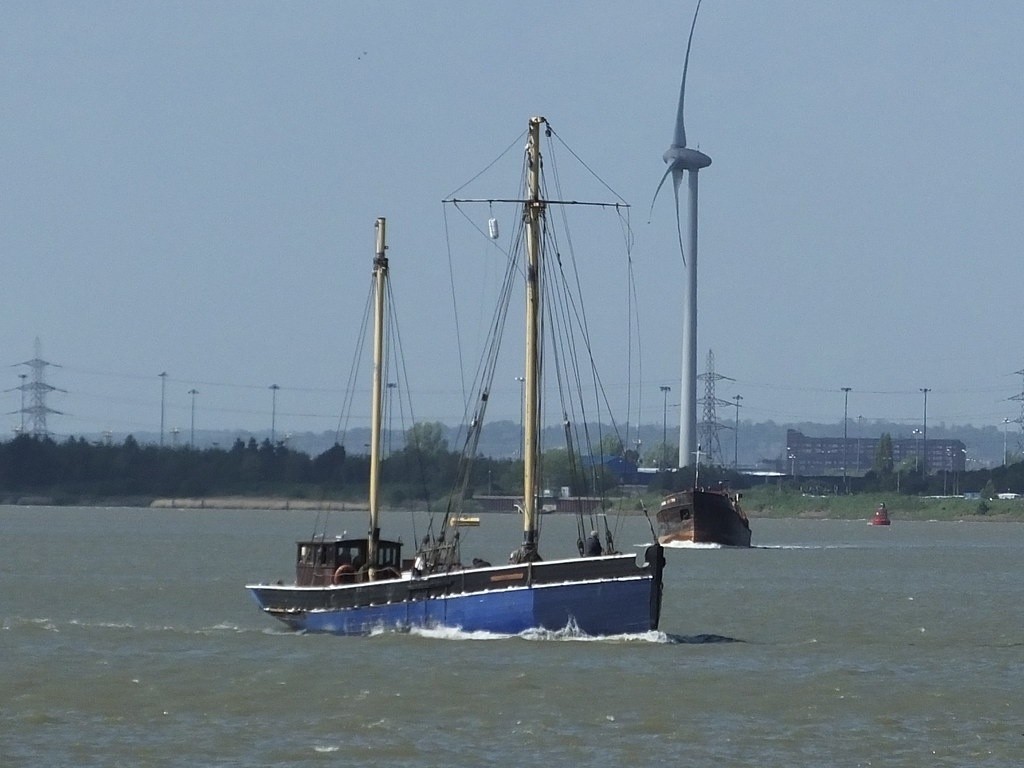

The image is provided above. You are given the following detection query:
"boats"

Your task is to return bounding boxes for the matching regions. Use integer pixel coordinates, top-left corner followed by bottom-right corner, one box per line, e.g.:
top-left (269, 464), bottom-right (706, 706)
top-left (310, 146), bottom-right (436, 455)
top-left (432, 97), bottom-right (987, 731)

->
top-left (653, 448), bottom-right (750, 552)
top-left (451, 517), bottom-right (483, 526)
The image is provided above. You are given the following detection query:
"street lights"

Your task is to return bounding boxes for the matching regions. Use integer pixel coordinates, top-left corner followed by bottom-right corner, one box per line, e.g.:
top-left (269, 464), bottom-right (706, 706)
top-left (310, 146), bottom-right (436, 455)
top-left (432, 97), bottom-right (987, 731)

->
top-left (269, 384), bottom-right (279, 444)
top-left (387, 382), bottom-right (397, 457)
top-left (1002, 418), bottom-right (1011, 465)
top-left (913, 429), bottom-right (923, 472)
top-left (788, 454), bottom-right (796, 476)
top-left (159, 372), bottom-right (171, 448)
top-left (857, 415), bottom-right (861, 473)
top-left (17, 374), bottom-right (28, 433)
top-left (659, 387), bottom-right (671, 467)
top-left (488, 470), bottom-right (491, 496)
top-left (188, 389), bottom-right (200, 448)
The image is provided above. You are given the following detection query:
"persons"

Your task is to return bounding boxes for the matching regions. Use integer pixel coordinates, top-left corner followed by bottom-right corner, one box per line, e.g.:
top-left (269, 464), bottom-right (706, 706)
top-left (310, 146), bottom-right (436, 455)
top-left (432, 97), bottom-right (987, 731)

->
top-left (411, 552), bottom-right (434, 578)
top-left (472, 558), bottom-right (491, 568)
top-left (584, 531), bottom-right (602, 557)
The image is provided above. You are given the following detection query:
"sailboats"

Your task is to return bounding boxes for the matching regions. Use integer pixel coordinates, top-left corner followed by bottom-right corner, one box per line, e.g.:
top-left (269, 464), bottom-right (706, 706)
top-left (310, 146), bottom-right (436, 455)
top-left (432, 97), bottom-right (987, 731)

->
top-left (242, 114), bottom-right (666, 637)
